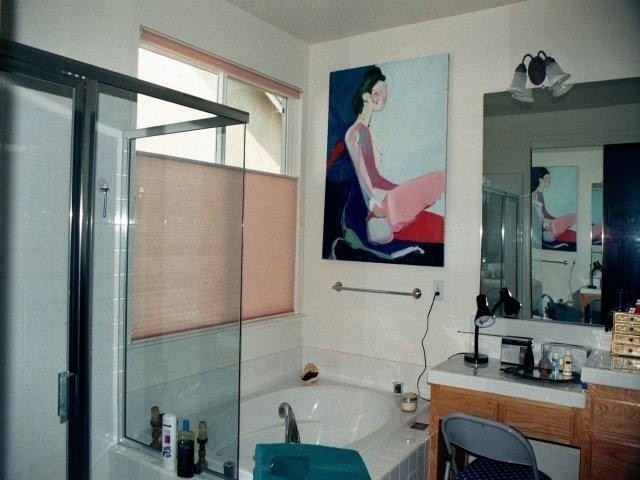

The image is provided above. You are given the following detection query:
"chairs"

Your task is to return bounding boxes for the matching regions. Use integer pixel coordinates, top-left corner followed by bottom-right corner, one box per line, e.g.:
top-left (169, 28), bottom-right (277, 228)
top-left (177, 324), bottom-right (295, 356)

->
top-left (433, 413), bottom-right (550, 480)
top-left (590, 299), bottom-right (599, 319)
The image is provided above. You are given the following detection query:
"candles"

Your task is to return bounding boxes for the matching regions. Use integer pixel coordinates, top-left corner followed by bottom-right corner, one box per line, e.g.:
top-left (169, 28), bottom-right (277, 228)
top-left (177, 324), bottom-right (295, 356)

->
top-left (199, 421), bottom-right (206, 441)
top-left (151, 406), bottom-right (159, 422)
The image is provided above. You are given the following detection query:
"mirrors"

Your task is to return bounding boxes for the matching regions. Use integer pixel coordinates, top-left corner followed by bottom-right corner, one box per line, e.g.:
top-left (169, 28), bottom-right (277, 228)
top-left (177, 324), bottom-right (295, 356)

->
top-left (530, 144), bottom-right (607, 326)
top-left (479, 76), bottom-right (640, 325)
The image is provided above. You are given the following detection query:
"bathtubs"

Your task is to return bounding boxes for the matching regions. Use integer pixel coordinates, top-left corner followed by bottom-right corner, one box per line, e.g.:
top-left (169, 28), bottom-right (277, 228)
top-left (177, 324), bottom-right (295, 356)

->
top-left (109, 374), bottom-right (431, 480)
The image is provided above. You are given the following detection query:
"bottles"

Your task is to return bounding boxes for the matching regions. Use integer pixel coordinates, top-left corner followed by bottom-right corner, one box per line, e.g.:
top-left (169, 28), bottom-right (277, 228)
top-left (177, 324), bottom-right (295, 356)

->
top-left (537, 349), bottom-right (552, 370)
top-left (551, 352), bottom-right (560, 374)
top-left (562, 349), bottom-right (573, 375)
top-left (523, 340), bottom-right (534, 371)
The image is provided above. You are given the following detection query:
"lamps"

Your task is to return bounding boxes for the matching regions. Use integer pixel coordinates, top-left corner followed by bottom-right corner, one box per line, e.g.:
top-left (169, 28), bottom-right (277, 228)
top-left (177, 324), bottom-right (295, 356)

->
top-left (489, 287), bottom-right (521, 316)
top-left (512, 82), bottom-right (574, 102)
top-left (587, 260), bottom-right (602, 287)
top-left (510, 51), bottom-right (571, 93)
top-left (464, 294), bottom-right (494, 368)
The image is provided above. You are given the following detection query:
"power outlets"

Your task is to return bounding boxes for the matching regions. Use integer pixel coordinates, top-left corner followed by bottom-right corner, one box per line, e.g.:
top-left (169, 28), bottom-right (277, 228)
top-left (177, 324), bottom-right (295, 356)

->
top-left (432, 280), bottom-right (444, 302)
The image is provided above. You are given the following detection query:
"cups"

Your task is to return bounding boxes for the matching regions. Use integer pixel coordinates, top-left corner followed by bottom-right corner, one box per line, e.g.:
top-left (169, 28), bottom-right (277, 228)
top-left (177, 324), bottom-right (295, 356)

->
top-left (392, 381), bottom-right (404, 396)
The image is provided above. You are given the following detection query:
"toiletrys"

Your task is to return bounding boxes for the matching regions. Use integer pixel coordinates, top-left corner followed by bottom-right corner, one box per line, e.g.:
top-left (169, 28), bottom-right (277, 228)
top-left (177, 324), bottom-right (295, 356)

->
top-left (537, 344), bottom-right (575, 379)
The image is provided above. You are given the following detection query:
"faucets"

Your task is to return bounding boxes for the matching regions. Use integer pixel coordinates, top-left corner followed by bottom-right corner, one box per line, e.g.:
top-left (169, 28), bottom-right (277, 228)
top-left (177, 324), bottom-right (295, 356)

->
top-left (542, 292), bottom-right (554, 304)
top-left (278, 401), bottom-right (301, 443)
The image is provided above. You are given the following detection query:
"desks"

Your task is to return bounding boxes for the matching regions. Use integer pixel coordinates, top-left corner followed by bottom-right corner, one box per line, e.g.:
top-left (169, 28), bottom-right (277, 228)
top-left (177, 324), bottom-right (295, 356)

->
top-left (579, 350), bottom-right (640, 480)
top-left (425, 352), bottom-right (587, 480)
top-left (580, 285), bottom-right (601, 324)
